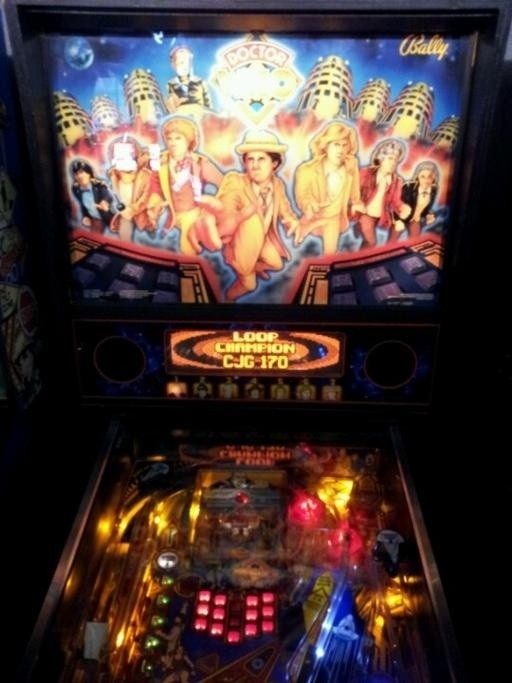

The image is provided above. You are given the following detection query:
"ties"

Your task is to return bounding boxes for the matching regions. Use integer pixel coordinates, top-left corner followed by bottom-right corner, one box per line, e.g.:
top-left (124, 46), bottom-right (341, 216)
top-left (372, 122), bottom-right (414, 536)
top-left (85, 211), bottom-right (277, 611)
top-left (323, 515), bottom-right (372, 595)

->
top-left (260, 188), bottom-right (269, 214)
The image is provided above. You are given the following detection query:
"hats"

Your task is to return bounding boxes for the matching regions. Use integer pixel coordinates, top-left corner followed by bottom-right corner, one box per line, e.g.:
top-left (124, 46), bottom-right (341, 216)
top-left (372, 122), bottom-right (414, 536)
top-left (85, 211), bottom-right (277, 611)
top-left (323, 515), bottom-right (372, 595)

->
top-left (235, 130), bottom-right (287, 154)
